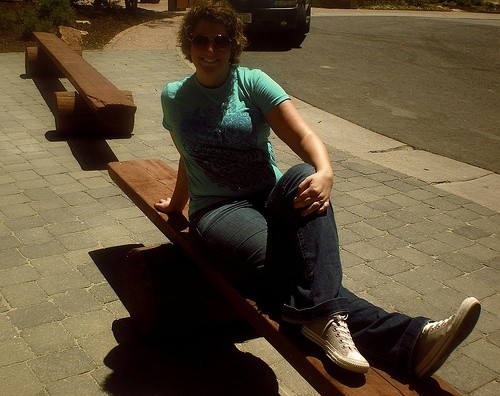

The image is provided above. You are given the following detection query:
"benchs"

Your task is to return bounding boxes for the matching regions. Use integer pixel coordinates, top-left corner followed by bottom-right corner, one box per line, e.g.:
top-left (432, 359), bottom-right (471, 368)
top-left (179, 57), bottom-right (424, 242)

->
top-left (24, 31), bottom-right (138, 137)
top-left (107, 159), bottom-right (465, 396)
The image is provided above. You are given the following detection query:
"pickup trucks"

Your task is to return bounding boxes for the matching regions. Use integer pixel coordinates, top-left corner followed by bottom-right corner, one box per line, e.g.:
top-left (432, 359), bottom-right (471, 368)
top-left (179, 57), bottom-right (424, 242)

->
top-left (229, 0), bottom-right (311, 47)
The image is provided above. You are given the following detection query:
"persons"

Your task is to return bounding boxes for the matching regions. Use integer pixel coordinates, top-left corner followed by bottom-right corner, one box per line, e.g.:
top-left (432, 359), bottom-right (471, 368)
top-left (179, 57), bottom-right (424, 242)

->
top-left (152, 0), bottom-right (482, 381)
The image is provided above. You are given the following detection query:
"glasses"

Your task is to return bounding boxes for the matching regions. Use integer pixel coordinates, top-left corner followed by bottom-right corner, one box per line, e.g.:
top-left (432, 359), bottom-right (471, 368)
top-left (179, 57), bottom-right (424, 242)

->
top-left (189, 33), bottom-right (233, 51)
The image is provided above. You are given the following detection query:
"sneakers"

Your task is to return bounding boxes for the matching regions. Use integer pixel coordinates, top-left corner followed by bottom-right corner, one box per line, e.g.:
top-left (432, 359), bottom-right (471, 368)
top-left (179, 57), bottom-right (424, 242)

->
top-left (413, 297), bottom-right (482, 380)
top-left (302, 312), bottom-right (370, 372)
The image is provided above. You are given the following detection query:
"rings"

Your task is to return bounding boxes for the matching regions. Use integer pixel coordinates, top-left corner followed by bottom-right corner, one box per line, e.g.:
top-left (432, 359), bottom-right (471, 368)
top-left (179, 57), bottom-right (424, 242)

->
top-left (315, 199), bottom-right (323, 206)
